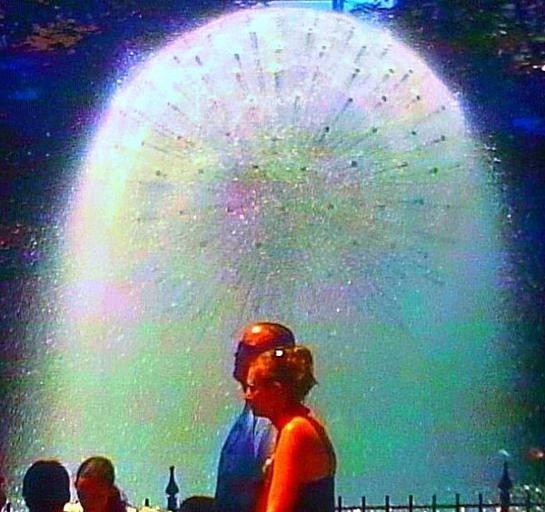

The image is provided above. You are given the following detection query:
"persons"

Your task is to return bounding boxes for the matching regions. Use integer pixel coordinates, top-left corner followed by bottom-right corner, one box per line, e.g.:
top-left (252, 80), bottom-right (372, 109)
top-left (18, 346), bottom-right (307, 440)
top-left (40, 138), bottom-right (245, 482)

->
top-left (243, 345), bottom-right (336, 512)
top-left (212, 323), bottom-right (296, 512)
top-left (22, 460), bottom-right (70, 511)
top-left (62, 456), bottom-right (137, 511)
